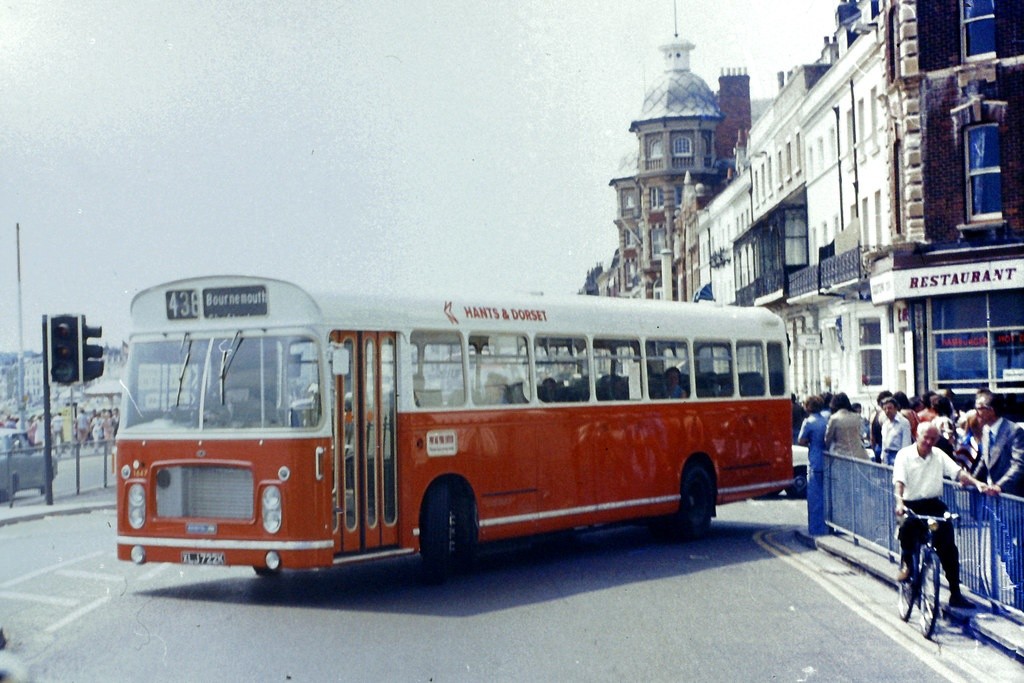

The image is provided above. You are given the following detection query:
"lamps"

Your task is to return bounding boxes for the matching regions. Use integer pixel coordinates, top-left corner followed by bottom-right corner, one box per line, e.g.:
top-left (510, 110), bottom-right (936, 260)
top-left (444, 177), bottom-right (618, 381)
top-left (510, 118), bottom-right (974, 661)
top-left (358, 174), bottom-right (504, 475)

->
top-left (850, 21), bottom-right (877, 36)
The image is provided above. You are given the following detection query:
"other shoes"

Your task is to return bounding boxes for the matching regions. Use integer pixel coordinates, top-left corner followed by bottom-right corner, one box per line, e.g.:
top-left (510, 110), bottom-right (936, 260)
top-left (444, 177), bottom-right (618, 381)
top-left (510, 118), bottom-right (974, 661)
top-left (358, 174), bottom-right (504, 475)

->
top-left (948, 596), bottom-right (977, 610)
top-left (899, 566), bottom-right (912, 583)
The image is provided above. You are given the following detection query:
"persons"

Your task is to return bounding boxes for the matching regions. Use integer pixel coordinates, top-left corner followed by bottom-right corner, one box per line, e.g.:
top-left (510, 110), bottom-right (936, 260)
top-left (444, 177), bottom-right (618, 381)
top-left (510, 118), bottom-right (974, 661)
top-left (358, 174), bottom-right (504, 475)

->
top-left (790, 389), bottom-right (1024, 615)
top-left (0, 407), bottom-right (119, 453)
top-left (414, 367), bottom-right (686, 413)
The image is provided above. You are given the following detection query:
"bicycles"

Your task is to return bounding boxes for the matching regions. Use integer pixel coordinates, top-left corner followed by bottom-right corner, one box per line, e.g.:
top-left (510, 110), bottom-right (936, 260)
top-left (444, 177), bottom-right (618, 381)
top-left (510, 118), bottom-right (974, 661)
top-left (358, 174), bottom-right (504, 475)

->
top-left (897, 506), bottom-right (959, 640)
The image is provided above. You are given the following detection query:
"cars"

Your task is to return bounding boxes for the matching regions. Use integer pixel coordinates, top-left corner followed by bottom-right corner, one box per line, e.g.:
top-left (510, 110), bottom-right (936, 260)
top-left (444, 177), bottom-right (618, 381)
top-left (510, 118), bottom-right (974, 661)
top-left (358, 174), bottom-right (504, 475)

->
top-left (787, 444), bottom-right (875, 497)
top-left (0, 431), bottom-right (59, 503)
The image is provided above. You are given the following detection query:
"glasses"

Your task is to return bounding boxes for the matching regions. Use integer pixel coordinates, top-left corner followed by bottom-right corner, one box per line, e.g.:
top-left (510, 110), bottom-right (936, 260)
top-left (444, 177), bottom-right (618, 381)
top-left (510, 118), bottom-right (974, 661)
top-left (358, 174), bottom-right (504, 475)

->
top-left (974, 407), bottom-right (988, 413)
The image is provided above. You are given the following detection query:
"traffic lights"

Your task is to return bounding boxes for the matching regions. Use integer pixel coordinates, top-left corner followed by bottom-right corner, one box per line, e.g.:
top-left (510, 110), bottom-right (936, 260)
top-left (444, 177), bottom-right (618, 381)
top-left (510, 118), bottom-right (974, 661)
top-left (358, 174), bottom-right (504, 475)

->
top-left (52, 316), bottom-right (80, 383)
top-left (82, 315), bottom-right (105, 382)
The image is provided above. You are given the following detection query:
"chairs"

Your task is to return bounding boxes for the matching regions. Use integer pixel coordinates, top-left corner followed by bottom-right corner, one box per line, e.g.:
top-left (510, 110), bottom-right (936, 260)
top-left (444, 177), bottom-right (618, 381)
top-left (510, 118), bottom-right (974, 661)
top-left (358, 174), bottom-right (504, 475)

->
top-left (290, 373), bottom-right (765, 430)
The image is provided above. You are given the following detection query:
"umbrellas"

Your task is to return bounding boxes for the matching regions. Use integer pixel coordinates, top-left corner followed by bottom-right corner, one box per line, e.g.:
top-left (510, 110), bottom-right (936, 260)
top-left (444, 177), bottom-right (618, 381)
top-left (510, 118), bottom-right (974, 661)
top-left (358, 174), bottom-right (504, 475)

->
top-left (86, 381), bottom-right (130, 407)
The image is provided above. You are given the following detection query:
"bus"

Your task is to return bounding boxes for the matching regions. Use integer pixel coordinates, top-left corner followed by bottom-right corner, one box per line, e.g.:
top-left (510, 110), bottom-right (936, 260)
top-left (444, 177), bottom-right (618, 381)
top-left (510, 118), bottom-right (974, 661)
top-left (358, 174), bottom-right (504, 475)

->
top-left (116, 276), bottom-right (793, 576)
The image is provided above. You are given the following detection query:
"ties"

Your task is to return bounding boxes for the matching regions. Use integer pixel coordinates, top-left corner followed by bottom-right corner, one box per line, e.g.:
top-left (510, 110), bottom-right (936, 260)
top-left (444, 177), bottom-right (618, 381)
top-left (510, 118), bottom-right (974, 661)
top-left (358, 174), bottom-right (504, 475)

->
top-left (988, 430), bottom-right (994, 464)
